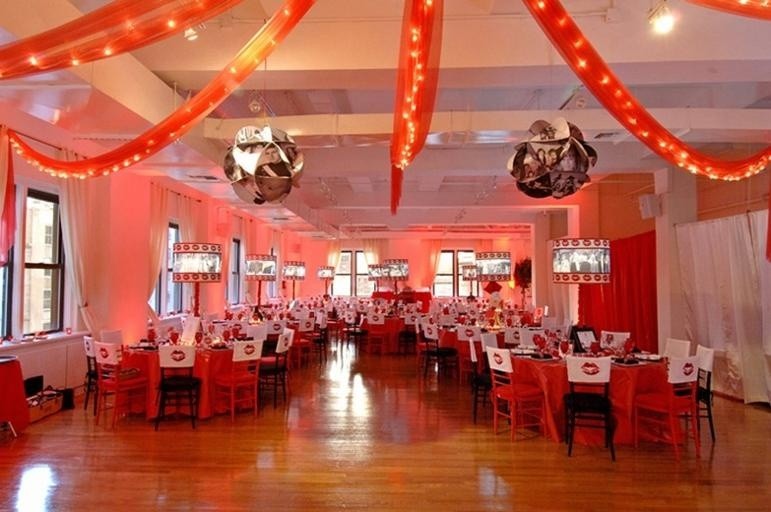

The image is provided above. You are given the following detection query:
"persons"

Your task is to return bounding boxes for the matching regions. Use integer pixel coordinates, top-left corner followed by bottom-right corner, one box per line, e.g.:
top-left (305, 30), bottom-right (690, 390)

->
top-left (554, 249), bottom-right (609, 273)
top-left (536, 149), bottom-right (546, 166)
top-left (256, 144), bottom-right (291, 176)
top-left (548, 149), bottom-right (558, 164)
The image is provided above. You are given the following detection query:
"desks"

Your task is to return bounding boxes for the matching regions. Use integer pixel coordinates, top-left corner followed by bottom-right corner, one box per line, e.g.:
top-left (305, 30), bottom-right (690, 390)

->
top-left (372, 290), bottom-right (432, 313)
top-left (0, 358), bottom-right (28, 438)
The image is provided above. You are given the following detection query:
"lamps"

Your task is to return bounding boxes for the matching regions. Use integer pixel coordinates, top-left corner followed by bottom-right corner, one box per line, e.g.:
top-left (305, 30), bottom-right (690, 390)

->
top-left (281, 261), bottom-right (305, 300)
top-left (318, 265), bottom-right (335, 298)
top-left (244, 254), bottom-right (277, 308)
top-left (367, 259), bottom-right (410, 296)
top-left (553, 237), bottom-right (611, 328)
top-left (508, 117), bottom-right (598, 200)
top-left (474, 252), bottom-right (513, 281)
top-left (173, 242), bottom-right (223, 316)
top-left (462, 265), bottom-right (478, 301)
top-left (222, 19), bottom-right (304, 203)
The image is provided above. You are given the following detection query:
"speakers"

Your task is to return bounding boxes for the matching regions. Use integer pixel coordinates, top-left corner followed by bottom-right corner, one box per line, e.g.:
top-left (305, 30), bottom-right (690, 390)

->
top-left (638, 194), bottom-right (661, 220)
